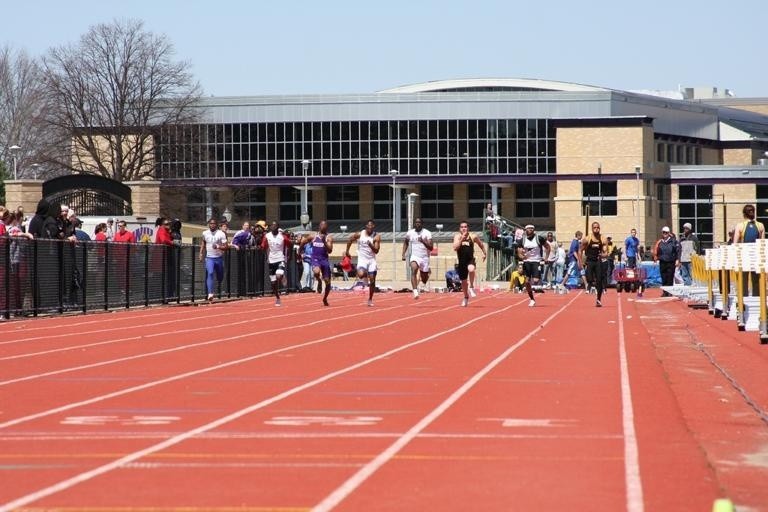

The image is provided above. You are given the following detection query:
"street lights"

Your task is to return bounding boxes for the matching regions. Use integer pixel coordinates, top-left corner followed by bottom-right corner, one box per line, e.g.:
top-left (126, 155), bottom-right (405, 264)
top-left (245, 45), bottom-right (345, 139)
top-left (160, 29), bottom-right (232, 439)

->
top-left (300, 206), bottom-right (310, 231)
top-left (407, 191), bottom-right (419, 281)
top-left (635, 164), bottom-right (641, 248)
top-left (9, 145), bottom-right (22, 180)
top-left (301, 159), bottom-right (312, 214)
top-left (388, 169), bottom-right (398, 291)
top-left (30, 163), bottom-right (41, 180)
top-left (222, 206), bottom-right (232, 230)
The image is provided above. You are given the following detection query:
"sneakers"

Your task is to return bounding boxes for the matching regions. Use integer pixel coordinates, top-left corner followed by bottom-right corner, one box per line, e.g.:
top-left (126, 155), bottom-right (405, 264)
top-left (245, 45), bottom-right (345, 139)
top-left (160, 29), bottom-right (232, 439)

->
top-left (596, 300), bottom-right (602, 307)
top-left (528, 300), bottom-right (536, 307)
top-left (281, 276), bottom-right (287, 287)
top-left (317, 281), bottom-right (322, 293)
top-left (275, 298), bottom-right (281, 307)
top-left (368, 301), bottom-right (374, 306)
top-left (414, 294), bottom-right (419, 300)
top-left (208, 294), bottom-right (214, 301)
top-left (468, 287), bottom-right (476, 298)
top-left (461, 294), bottom-right (469, 307)
top-left (322, 298), bottom-right (328, 306)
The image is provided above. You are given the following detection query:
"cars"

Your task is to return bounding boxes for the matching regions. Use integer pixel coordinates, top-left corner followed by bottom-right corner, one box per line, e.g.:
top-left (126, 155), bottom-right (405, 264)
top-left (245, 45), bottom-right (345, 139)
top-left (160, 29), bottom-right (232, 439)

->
top-left (331, 262), bottom-right (358, 278)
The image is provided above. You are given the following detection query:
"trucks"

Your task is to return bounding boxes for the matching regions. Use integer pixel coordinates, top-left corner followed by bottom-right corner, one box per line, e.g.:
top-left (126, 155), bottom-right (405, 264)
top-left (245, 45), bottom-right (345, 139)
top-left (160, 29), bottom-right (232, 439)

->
top-left (4, 214), bottom-right (161, 294)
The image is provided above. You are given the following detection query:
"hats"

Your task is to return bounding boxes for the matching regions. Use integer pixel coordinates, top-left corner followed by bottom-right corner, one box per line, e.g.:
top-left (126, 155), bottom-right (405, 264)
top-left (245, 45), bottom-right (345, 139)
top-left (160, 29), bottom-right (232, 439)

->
top-left (661, 226), bottom-right (670, 232)
top-left (683, 222), bottom-right (691, 230)
top-left (255, 220), bottom-right (266, 230)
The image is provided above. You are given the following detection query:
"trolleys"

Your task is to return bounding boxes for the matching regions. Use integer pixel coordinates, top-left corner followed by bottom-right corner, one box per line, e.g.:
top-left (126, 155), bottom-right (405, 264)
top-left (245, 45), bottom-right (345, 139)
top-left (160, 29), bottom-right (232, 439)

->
top-left (614, 248), bottom-right (647, 293)
